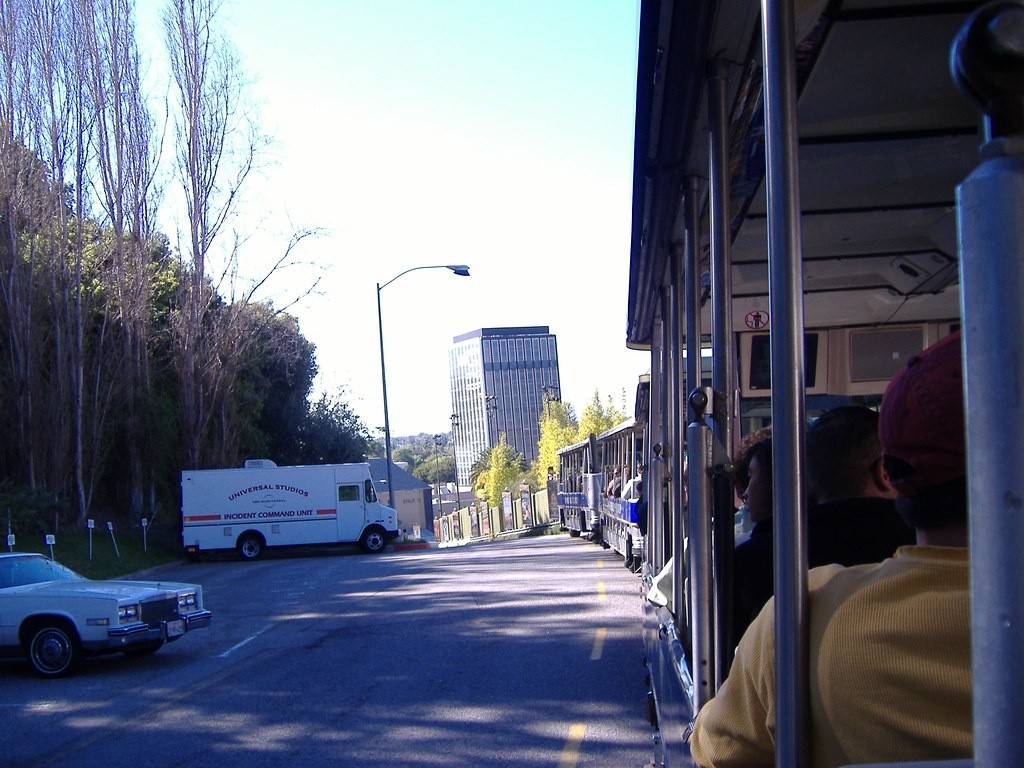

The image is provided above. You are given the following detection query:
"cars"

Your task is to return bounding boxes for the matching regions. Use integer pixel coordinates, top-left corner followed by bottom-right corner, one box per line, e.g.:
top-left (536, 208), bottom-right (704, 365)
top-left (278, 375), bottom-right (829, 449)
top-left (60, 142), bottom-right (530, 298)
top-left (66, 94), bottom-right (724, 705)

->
top-left (1, 552), bottom-right (214, 679)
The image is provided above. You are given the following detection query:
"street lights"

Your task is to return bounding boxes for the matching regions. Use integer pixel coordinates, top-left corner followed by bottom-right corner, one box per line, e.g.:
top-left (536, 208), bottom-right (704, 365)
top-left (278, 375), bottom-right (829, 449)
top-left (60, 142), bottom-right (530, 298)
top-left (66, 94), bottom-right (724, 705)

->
top-left (374, 263), bottom-right (471, 507)
top-left (541, 384), bottom-right (560, 419)
top-left (431, 434), bottom-right (442, 518)
top-left (484, 394), bottom-right (499, 449)
top-left (448, 414), bottom-right (461, 511)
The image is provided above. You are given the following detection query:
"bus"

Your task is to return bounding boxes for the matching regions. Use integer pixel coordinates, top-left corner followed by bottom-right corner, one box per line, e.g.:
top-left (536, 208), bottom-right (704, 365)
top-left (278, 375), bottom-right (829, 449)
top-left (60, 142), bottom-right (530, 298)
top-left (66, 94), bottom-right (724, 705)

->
top-left (558, 416), bottom-right (646, 573)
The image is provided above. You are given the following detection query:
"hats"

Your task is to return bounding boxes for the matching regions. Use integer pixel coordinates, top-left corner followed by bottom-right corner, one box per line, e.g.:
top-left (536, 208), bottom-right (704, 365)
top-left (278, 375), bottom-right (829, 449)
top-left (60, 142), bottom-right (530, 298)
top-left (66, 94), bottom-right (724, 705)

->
top-left (878, 329), bottom-right (965, 494)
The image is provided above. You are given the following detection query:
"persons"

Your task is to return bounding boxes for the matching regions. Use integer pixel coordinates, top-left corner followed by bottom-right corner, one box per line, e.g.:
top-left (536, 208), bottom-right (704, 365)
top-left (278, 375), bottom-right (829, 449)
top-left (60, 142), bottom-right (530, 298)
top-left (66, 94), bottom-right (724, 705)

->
top-left (572, 404), bottom-right (914, 684)
top-left (691, 329), bottom-right (975, 768)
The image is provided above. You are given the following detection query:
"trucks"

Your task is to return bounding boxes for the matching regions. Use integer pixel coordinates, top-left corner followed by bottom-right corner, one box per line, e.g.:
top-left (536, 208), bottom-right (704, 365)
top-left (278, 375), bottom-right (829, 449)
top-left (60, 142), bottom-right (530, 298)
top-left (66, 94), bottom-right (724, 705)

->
top-left (180, 458), bottom-right (398, 562)
